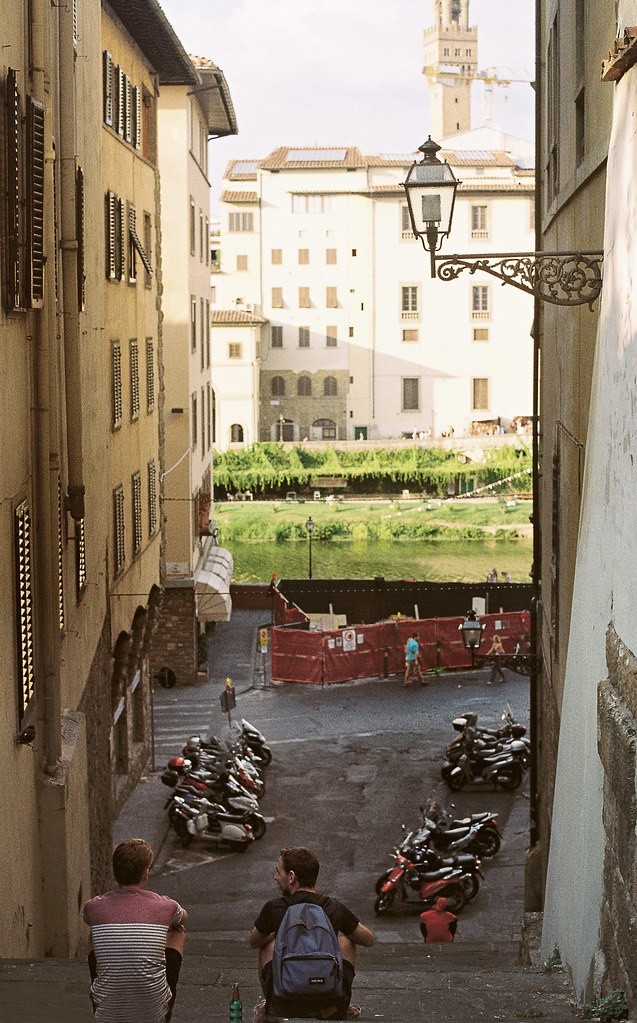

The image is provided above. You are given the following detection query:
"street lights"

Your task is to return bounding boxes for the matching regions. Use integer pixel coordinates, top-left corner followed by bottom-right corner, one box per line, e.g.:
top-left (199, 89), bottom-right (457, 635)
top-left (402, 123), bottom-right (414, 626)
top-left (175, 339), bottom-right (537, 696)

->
top-left (305, 516), bottom-right (314, 579)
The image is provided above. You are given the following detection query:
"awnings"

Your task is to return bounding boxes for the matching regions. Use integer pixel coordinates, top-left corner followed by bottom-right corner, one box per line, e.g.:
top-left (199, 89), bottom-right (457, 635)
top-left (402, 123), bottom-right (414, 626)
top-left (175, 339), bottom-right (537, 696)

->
top-left (196, 545), bottom-right (234, 622)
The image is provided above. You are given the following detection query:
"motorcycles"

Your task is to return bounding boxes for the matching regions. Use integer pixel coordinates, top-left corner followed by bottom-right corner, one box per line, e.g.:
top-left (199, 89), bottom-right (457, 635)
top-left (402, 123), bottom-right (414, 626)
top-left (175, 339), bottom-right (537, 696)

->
top-left (440, 714), bottom-right (532, 793)
top-left (160, 717), bottom-right (272, 852)
top-left (373, 789), bottom-right (504, 916)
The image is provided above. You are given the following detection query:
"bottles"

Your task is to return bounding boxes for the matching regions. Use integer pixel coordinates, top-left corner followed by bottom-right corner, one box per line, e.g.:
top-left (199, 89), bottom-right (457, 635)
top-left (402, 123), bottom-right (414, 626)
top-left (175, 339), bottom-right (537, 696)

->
top-left (229, 982), bottom-right (242, 1023)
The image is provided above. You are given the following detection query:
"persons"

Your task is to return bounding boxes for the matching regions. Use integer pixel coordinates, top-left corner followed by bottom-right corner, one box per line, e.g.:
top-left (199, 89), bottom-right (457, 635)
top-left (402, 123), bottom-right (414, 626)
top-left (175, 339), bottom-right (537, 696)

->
top-left (419, 898), bottom-right (458, 943)
top-left (485, 635), bottom-right (506, 685)
top-left (83, 838), bottom-right (188, 1023)
top-left (403, 633), bottom-right (430, 685)
top-left (250, 846), bottom-right (375, 1023)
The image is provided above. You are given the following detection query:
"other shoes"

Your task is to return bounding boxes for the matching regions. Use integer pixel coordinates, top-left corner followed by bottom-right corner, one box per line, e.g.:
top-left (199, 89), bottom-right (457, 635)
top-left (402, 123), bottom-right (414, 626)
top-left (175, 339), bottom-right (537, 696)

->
top-left (422, 680), bottom-right (430, 684)
top-left (254, 998), bottom-right (268, 1014)
top-left (344, 1004), bottom-right (361, 1019)
top-left (403, 683), bottom-right (411, 687)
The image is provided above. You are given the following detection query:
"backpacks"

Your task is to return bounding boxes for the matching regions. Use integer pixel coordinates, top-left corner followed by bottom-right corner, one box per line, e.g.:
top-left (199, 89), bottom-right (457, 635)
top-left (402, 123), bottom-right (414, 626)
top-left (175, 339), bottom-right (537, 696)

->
top-left (271, 893), bottom-right (343, 1003)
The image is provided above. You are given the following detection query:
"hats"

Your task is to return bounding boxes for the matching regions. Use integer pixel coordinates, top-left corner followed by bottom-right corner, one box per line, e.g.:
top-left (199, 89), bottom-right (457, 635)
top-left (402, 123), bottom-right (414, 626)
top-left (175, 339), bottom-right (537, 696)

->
top-left (435, 897), bottom-right (449, 911)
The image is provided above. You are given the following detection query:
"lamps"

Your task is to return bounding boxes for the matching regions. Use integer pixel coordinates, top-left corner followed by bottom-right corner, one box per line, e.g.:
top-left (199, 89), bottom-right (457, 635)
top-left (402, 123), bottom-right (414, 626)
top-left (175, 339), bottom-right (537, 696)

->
top-left (398, 134), bottom-right (603, 314)
top-left (461, 610), bottom-right (532, 676)
